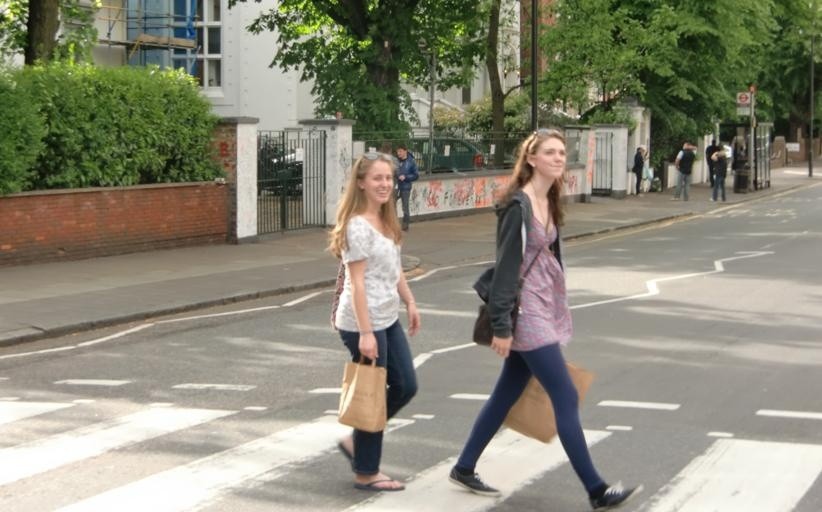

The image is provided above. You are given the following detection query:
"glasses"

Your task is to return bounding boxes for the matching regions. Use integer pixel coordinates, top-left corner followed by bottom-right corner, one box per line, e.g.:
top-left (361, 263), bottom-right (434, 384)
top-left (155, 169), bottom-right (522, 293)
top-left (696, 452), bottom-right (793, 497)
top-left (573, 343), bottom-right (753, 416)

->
top-left (364, 152), bottom-right (393, 161)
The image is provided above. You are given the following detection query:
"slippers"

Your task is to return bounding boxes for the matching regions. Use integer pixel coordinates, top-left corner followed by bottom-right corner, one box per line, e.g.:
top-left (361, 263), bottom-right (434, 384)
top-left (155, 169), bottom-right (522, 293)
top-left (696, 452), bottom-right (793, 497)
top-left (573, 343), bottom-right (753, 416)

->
top-left (338, 440), bottom-right (352, 459)
top-left (353, 478), bottom-right (405, 491)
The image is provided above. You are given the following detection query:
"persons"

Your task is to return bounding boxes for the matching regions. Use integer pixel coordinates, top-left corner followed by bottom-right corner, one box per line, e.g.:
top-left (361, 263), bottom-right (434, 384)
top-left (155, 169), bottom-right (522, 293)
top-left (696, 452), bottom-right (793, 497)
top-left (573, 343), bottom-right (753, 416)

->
top-left (449, 128), bottom-right (645, 512)
top-left (706, 140), bottom-right (720, 186)
top-left (393, 144), bottom-right (420, 232)
top-left (710, 151), bottom-right (729, 202)
top-left (632, 147), bottom-right (645, 197)
top-left (328, 152), bottom-right (419, 492)
top-left (670, 142), bottom-right (698, 202)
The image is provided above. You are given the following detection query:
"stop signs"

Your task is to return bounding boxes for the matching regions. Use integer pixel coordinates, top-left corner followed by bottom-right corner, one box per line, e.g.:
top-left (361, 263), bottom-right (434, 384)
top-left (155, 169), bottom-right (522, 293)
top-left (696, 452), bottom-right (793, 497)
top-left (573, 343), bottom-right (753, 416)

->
top-left (738, 93), bottom-right (748, 102)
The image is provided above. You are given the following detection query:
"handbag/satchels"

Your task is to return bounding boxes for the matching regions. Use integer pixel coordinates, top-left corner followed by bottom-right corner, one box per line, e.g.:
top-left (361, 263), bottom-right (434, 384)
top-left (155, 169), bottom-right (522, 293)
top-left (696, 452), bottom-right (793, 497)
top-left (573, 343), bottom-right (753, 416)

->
top-left (472, 295), bottom-right (521, 347)
top-left (337, 355), bottom-right (388, 433)
top-left (503, 347), bottom-right (595, 444)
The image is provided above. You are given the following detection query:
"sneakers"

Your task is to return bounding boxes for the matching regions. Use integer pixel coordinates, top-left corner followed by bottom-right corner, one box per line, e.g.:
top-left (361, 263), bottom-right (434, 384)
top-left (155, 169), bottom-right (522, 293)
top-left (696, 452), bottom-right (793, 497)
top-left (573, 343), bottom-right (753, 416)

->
top-left (448, 466), bottom-right (502, 497)
top-left (588, 481), bottom-right (644, 512)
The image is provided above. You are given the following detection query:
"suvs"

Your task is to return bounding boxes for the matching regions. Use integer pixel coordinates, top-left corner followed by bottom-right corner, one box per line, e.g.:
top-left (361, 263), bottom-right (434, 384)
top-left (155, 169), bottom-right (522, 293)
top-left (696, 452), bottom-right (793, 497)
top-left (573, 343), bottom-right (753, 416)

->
top-left (393, 137), bottom-right (486, 173)
